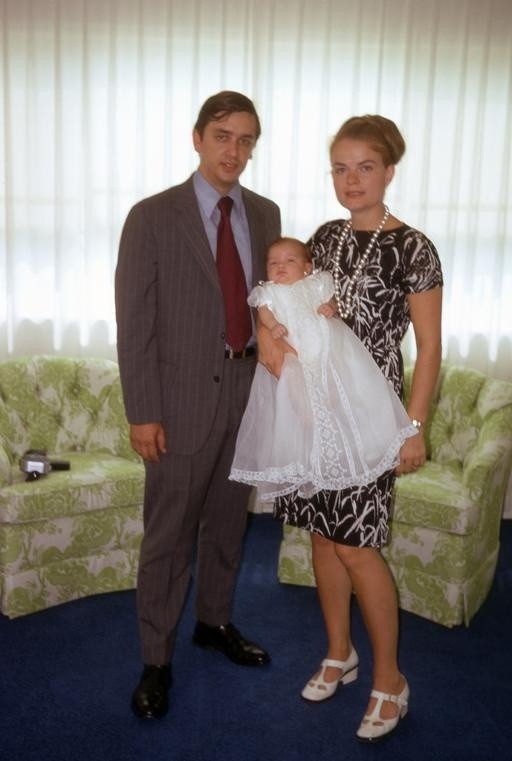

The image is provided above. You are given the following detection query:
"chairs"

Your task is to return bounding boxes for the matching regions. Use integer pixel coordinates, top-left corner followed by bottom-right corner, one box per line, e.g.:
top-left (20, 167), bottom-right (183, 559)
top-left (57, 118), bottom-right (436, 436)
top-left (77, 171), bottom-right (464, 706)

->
top-left (1, 354), bottom-right (146, 619)
top-left (277, 364), bottom-right (511, 627)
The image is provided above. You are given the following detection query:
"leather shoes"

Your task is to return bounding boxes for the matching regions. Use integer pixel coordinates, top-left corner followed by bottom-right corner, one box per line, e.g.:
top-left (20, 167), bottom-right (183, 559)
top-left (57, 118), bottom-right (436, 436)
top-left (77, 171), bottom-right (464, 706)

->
top-left (191, 618), bottom-right (271, 669)
top-left (131, 664), bottom-right (170, 720)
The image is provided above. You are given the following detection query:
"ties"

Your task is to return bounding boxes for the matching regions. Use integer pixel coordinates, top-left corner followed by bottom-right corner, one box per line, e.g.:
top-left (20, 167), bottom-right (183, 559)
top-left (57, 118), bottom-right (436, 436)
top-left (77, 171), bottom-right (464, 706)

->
top-left (214, 195), bottom-right (253, 354)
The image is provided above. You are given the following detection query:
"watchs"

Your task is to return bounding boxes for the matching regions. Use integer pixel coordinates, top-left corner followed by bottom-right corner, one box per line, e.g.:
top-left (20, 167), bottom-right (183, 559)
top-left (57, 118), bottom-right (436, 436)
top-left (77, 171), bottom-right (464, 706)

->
top-left (408, 419), bottom-right (425, 430)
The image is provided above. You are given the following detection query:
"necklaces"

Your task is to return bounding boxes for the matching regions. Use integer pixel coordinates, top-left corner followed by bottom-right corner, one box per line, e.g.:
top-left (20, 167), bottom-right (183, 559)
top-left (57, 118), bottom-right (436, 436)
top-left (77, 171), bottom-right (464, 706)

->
top-left (334, 205), bottom-right (389, 322)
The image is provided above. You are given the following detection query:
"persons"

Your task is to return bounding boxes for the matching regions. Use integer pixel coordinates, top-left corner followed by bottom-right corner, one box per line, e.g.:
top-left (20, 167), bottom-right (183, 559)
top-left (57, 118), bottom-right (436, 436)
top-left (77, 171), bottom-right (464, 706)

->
top-left (114, 91), bottom-right (274, 718)
top-left (227, 238), bottom-right (420, 503)
top-left (255, 114), bottom-right (443, 744)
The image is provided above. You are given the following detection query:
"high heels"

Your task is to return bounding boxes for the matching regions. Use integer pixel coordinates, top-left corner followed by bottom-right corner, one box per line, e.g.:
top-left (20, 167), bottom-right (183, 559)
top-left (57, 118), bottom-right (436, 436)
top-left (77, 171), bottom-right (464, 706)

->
top-left (355, 672), bottom-right (411, 743)
top-left (300, 647), bottom-right (361, 702)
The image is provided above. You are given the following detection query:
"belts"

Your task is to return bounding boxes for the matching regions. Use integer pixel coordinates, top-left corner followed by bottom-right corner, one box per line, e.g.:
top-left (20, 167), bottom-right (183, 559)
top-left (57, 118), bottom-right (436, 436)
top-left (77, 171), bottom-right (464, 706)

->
top-left (226, 347), bottom-right (257, 359)
